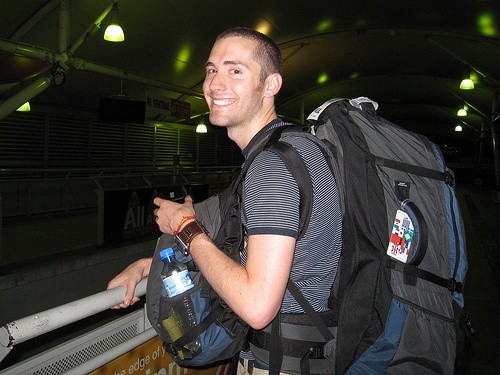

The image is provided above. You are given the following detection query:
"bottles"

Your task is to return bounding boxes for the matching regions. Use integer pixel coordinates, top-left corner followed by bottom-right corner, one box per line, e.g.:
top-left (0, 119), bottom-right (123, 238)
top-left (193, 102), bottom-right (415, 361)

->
top-left (161, 315), bottom-right (189, 360)
top-left (159, 247), bottom-right (201, 354)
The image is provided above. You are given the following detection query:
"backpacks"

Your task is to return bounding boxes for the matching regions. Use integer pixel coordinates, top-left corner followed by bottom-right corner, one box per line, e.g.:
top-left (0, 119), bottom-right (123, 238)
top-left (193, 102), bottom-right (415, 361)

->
top-left (146, 141), bottom-right (315, 371)
top-left (241, 96), bottom-right (468, 374)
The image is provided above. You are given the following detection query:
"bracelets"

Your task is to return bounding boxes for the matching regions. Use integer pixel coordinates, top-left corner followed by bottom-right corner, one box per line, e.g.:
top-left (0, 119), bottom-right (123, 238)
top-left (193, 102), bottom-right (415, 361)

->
top-left (174, 215), bottom-right (197, 236)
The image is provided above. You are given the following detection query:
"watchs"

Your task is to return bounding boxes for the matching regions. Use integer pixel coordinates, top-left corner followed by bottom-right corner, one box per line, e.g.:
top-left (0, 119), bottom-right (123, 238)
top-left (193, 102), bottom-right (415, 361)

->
top-left (174, 221), bottom-right (210, 257)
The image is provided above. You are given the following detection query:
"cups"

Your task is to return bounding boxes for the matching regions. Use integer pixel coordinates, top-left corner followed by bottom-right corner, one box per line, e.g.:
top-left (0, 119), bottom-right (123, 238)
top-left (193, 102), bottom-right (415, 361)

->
top-left (160, 184), bottom-right (187, 205)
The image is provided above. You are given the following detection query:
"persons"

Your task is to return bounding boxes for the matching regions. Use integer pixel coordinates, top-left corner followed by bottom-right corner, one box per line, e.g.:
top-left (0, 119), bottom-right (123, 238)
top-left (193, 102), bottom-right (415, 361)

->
top-left (107, 28), bottom-right (343, 375)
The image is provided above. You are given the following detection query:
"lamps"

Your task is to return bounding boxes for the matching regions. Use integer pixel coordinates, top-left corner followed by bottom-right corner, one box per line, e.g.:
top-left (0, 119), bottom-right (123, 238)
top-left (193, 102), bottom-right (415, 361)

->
top-left (455, 70), bottom-right (494, 133)
top-left (195, 116), bottom-right (207, 133)
top-left (104, 4), bottom-right (125, 42)
